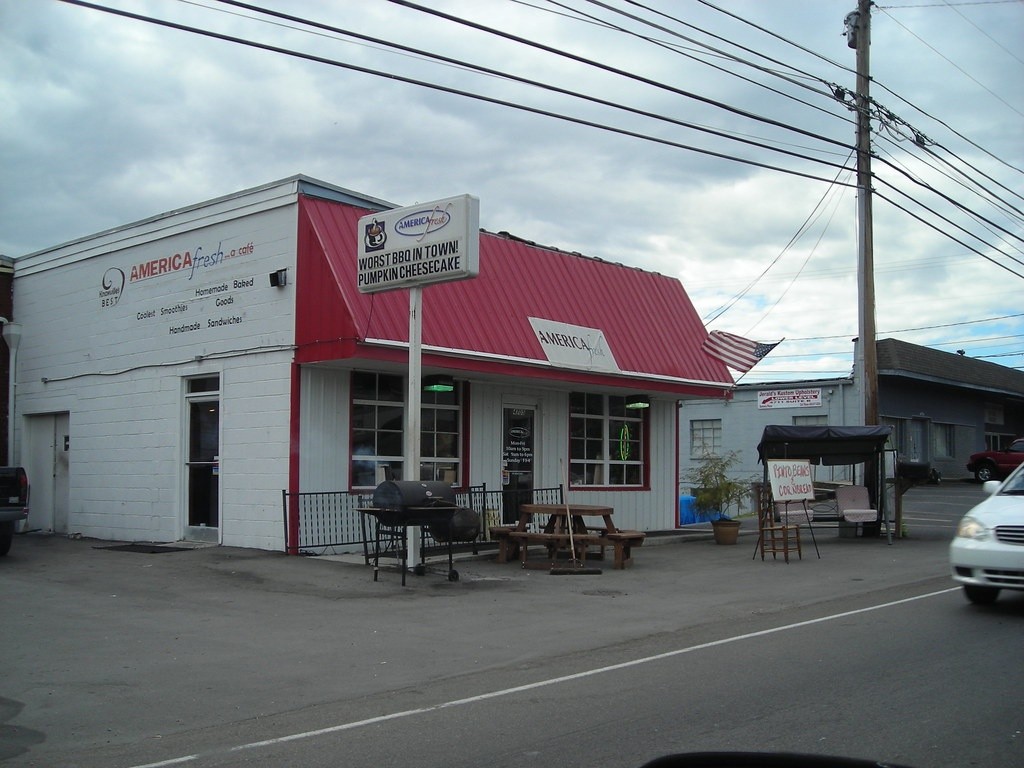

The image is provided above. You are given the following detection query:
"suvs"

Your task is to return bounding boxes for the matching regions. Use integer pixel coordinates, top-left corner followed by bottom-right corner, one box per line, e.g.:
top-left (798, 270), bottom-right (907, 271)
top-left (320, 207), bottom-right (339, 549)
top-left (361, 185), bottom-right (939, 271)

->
top-left (966, 438), bottom-right (1024, 483)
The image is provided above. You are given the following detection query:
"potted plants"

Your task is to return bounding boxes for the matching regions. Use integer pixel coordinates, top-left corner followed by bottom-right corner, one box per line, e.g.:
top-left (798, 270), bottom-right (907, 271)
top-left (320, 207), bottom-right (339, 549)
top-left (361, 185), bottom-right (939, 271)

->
top-left (677, 429), bottom-right (764, 544)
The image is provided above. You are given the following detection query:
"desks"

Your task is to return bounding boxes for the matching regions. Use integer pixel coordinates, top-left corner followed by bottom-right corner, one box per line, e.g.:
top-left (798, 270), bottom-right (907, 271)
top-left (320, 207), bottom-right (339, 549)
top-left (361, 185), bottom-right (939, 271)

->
top-left (505, 504), bottom-right (631, 564)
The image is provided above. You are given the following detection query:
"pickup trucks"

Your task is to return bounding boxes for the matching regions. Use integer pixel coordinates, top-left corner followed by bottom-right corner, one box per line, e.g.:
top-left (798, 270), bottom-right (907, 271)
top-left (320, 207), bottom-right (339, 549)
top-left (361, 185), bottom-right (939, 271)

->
top-left (0, 465), bottom-right (32, 555)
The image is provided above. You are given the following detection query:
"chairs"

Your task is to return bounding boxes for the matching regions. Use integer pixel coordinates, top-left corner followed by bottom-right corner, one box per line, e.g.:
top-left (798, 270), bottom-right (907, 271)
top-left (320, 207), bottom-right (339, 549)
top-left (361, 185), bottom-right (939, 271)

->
top-left (752, 481), bottom-right (803, 565)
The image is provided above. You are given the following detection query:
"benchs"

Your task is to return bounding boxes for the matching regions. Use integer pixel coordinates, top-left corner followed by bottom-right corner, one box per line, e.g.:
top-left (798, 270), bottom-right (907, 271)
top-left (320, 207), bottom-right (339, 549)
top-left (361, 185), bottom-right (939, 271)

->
top-left (490, 525), bottom-right (646, 570)
top-left (767, 483), bottom-right (879, 538)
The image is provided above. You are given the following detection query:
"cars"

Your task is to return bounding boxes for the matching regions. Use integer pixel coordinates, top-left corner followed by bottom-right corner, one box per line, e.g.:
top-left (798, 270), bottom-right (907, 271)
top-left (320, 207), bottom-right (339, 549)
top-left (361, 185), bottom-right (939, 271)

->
top-left (948, 463), bottom-right (1024, 604)
